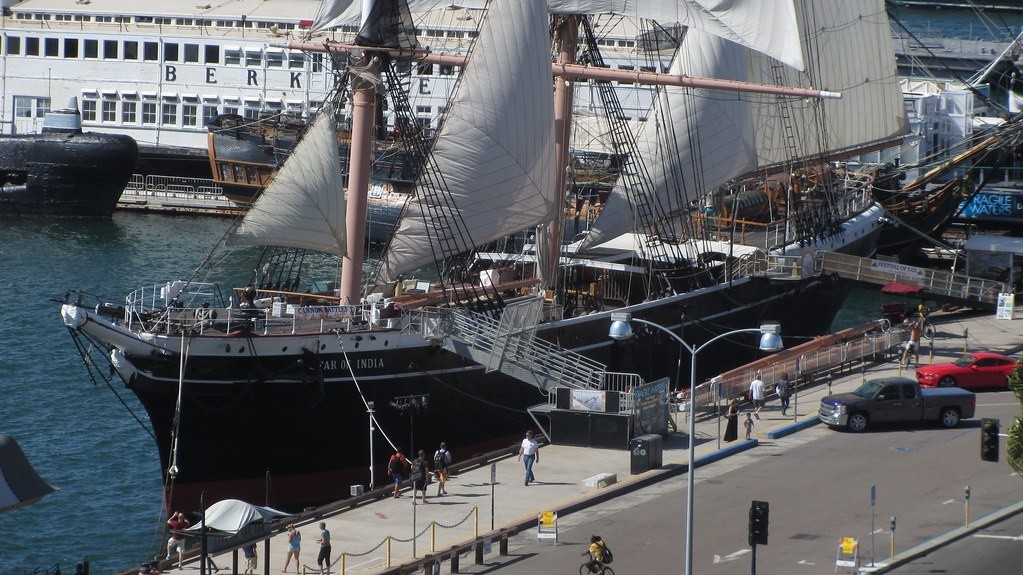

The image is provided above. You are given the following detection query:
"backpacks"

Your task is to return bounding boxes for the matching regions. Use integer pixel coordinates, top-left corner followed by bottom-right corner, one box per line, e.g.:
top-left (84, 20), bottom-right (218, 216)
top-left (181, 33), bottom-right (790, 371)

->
top-left (595, 540), bottom-right (613, 564)
top-left (434, 450), bottom-right (448, 469)
top-left (390, 454), bottom-right (403, 474)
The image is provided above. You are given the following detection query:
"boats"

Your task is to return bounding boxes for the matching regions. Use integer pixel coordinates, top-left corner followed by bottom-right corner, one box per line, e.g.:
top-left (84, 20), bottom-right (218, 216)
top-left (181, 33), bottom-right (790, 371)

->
top-left (0, 95), bottom-right (141, 218)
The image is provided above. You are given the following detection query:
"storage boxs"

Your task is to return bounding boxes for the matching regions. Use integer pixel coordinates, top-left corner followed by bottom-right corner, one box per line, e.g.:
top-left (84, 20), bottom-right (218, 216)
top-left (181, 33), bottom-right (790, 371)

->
top-left (255, 297), bottom-right (301, 317)
top-left (479, 270), bottom-right (500, 290)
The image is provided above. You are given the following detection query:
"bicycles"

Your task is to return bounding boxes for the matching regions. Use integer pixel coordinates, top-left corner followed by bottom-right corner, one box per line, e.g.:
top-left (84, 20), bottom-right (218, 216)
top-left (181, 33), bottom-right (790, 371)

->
top-left (579, 552), bottom-right (615, 575)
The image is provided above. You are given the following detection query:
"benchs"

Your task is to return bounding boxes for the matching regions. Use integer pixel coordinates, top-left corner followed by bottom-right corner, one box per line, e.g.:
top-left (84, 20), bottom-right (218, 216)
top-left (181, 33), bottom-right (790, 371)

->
top-left (576, 473), bottom-right (616, 494)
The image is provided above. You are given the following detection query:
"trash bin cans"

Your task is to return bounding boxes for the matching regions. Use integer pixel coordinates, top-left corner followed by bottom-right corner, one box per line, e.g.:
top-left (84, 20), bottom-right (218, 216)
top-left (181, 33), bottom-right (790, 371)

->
top-left (641, 434), bottom-right (662, 468)
top-left (630, 436), bottom-right (656, 475)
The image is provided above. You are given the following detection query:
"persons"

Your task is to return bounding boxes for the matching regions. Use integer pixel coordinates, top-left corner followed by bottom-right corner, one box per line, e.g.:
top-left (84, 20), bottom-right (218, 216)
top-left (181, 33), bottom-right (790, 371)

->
top-left (724, 399), bottom-right (740, 442)
top-left (749, 375), bottom-right (767, 419)
top-left (316, 522), bottom-right (331, 575)
top-left (193, 285), bottom-right (257, 321)
top-left (242, 542), bottom-right (258, 575)
top-left (518, 430), bottom-right (539, 486)
top-left (410, 450), bottom-right (429, 505)
top-left (743, 413), bottom-right (755, 440)
top-left (433, 442), bottom-right (451, 497)
top-left (167, 512), bottom-right (190, 570)
top-left (581, 535), bottom-right (613, 575)
top-left (281, 523), bottom-right (301, 575)
top-left (388, 447), bottom-right (409, 498)
top-left (901, 297), bottom-right (931, 368)
top-left (777, 373), bottom-right (792, 415)
top-left (207, 557), bottom-right (219, 575)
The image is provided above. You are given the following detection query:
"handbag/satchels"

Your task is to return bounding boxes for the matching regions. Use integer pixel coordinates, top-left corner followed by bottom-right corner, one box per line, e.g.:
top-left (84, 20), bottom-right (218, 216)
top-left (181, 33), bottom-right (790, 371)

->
top-left (724, 410), bottom-right (733, 420)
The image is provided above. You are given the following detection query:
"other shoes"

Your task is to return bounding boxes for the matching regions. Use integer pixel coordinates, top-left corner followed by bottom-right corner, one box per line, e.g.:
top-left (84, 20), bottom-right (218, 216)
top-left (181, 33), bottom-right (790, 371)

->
top-left (280, 568), bottom-right (286, 572)
top-left (423, 500), bottom-right (428, 503)
top-left (397, 493), bottom-right (401, 496)
top-left (593, 572), bottom-right (598, 575)
top-left (531, 478), bottom-right (534, 482)
top-left (412, 501), bottom-right (416, 505)
top-left (782, 409), bottom-right (785, 415)
top-left (295, 572), bottom-right (300, 574)
top-left (525, 481), bottom-right (528, 486)
top-left (394, 496), bottom-right (400, 498)
top-left (442, 491), bottom-right (447, 494)
top-left (437, 493), bottom-right (443, 496)
top-left (754, 413), bottom-right (759, 419)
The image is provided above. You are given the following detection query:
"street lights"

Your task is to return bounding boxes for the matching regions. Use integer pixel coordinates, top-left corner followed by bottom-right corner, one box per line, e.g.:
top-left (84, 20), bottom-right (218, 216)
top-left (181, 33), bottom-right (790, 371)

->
top-left (389, 393), bottom-right (431, 478)
top-left (366, 401), bottom-right (377, 492)
top-left (606, 309), bottom-right (783, 575)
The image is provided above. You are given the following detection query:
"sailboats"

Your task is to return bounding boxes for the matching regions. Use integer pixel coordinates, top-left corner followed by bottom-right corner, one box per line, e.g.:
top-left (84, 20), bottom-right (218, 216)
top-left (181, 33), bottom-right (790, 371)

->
top-left (204, 1), bottom-right (1023, 258)
top-left (50, 0), bottom-right (922, 530)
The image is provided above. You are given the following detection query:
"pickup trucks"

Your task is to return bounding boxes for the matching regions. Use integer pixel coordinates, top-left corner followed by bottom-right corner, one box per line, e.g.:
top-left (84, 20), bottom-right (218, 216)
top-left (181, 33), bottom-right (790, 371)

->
top-left (818, 377), bottom-right (976, 433)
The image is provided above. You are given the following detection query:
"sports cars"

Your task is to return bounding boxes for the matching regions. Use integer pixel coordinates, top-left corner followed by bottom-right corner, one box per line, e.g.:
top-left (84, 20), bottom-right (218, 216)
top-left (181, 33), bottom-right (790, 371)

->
top-left (914, 352), bottom-right (1023, 392)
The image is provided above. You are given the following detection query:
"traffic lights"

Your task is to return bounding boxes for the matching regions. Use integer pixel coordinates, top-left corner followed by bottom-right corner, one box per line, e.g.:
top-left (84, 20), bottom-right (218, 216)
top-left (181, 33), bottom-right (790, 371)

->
top-left (981, 417), bottom-right (1001, 463)
top-left (752, 501), bottom-right (769, 545)
